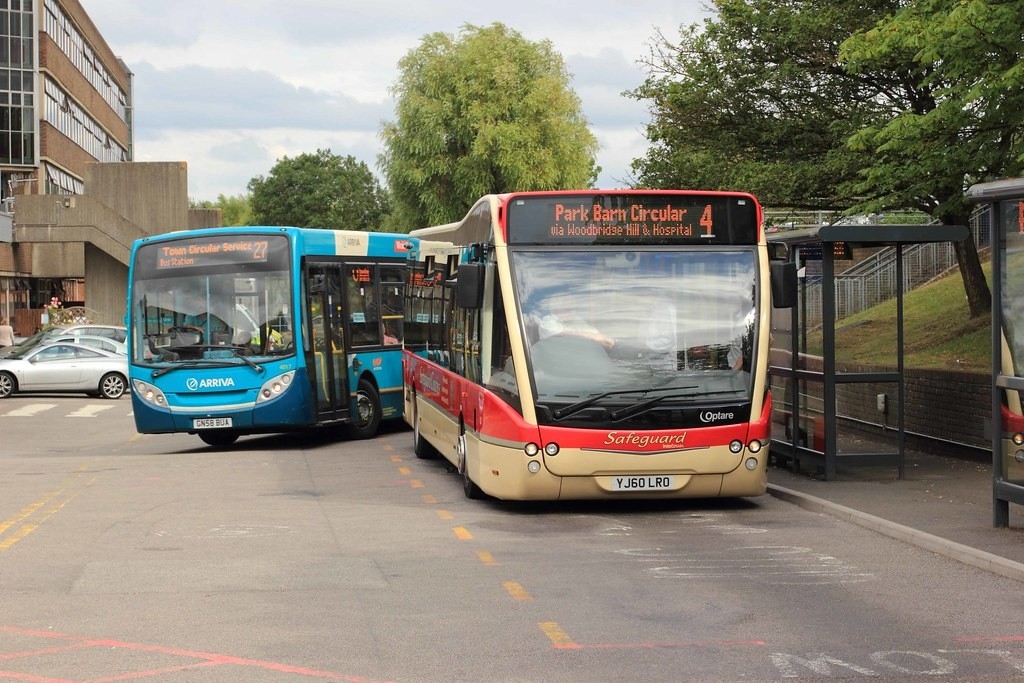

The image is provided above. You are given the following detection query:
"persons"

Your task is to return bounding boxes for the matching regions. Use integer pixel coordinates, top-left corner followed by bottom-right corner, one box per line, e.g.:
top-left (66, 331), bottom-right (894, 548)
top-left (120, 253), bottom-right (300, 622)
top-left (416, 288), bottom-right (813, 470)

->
top-left (0, 318), bottom-right (15, 348)
top-left (641, 285), bottom-right (677, 372)
top-left (538, 293), bottom-right (614, 350)
top-left (726, 291), bottom-right (774, 371)
top-left (185, 295), bottom-right (220, 341)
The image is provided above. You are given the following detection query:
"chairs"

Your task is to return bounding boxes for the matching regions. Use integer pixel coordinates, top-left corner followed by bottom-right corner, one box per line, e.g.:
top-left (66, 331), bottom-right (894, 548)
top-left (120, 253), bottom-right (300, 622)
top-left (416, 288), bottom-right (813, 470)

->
top-left (56, 349), bottom-right (68, 359)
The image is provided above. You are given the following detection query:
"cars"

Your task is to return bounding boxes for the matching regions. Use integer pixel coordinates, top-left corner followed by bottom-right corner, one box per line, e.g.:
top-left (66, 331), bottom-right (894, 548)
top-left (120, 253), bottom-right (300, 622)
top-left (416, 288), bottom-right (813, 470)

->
top-left (0, 324), bottom-right (130, 399)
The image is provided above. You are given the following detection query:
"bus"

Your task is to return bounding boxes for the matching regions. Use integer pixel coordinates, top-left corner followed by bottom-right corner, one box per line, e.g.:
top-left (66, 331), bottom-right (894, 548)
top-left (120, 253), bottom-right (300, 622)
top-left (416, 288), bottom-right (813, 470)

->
top-left (401, 189), bottom-right (799, 506)
top-left (127, 217), bottom-right (487, 446)
